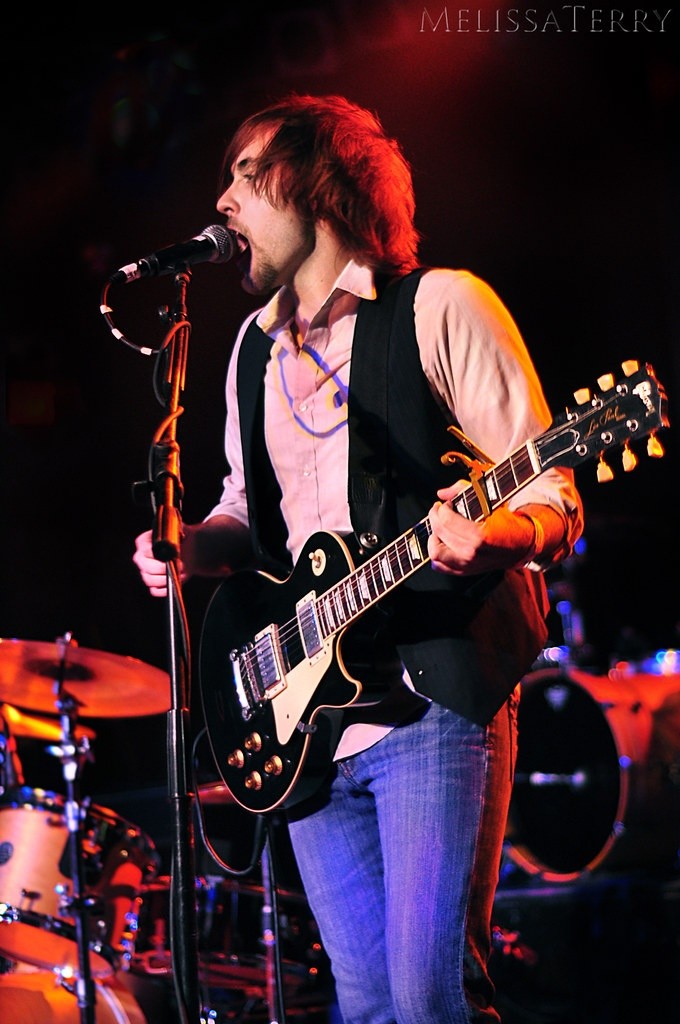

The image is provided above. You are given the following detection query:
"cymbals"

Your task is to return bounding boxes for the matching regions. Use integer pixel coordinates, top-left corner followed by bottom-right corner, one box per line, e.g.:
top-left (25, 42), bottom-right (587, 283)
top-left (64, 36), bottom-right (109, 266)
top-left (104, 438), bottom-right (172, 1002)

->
top-left (0, 637), bottom-right (171, 718)
top-left (0, 702), bottom-right (96, 743)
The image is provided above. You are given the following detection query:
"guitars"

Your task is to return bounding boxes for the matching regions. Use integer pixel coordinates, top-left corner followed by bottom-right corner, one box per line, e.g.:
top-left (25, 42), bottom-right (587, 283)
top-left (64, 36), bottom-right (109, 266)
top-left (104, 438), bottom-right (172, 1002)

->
top-left (201, 360), bottom-right (669, 817)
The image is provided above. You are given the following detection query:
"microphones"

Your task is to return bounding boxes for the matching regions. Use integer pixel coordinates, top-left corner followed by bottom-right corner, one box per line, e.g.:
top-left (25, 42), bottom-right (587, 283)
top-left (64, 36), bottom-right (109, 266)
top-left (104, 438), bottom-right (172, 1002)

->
top-left (110, 224), bottom-right (239, 285)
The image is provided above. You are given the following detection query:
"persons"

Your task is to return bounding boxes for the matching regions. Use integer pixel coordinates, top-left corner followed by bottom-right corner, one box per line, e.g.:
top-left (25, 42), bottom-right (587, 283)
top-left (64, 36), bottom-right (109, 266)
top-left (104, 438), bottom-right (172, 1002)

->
top-left (133, 93), bottom-right (585, 1024)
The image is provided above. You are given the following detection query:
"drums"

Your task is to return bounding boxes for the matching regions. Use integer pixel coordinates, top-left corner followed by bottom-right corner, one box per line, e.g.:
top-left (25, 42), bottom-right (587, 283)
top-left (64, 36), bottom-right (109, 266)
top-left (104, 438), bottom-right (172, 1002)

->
top-left (502, 669), bottom-right (679, 882)
top-left (124, 867), bottom-right (307, 986)
top-left (0, 784), bottom-right (159, 981)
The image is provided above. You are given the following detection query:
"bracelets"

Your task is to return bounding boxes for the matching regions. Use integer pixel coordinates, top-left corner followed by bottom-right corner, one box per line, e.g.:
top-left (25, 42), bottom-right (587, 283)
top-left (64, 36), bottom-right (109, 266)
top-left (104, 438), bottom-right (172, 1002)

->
top-left (518, 510), bottom-right (546, 566)
